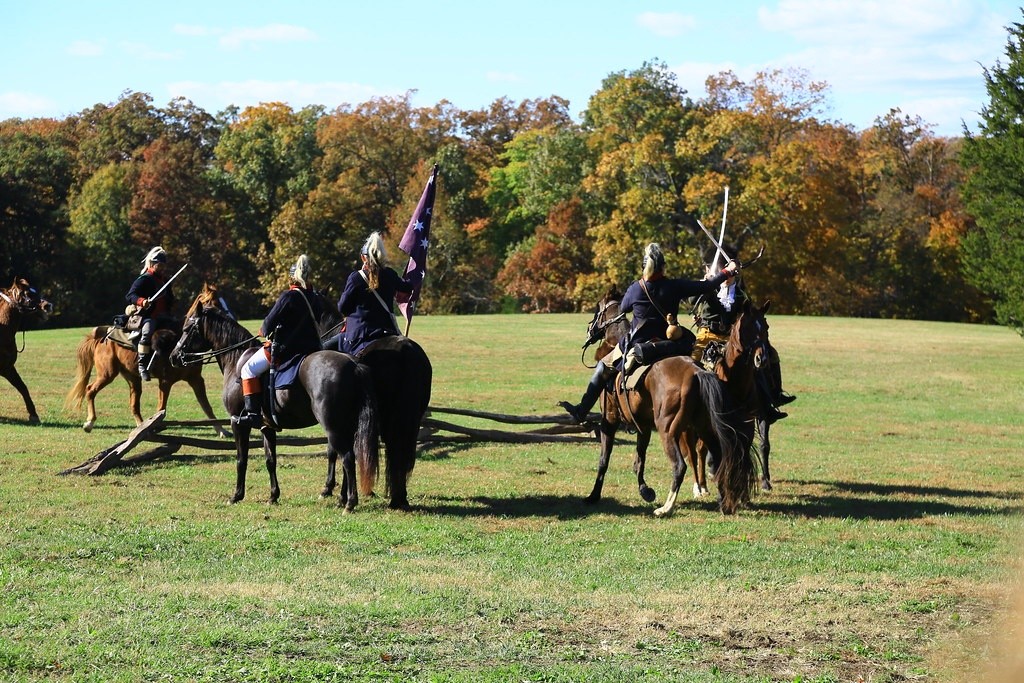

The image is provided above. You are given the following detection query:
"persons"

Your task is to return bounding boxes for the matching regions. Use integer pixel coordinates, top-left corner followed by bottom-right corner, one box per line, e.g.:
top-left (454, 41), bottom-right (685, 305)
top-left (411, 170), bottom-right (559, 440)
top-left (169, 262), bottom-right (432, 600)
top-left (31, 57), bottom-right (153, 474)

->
top-left (230, 254), bottom-right (324, 425)
top-left (692, 247), bottom-right (796, 425)
top-left (123, 246), bottom-right (186, 381)
top-left (335, 229), bottom-right (412, 354)
top-left (556, 242), bottom-right (739, 424)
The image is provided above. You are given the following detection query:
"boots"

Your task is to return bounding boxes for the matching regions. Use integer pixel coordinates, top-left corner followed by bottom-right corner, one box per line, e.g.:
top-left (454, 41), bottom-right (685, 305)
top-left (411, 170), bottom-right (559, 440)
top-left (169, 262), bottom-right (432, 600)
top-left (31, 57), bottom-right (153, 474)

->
top-left (754, 369), bottom-right (787, 421)
top-left (230, 376), bottom-right (263, 427)
top-left (563, 382), bottom-right (603, 423)
top-left (766, 362), bottom-right (795, 407)
top-left (136, 344), bottom-right (151, 381)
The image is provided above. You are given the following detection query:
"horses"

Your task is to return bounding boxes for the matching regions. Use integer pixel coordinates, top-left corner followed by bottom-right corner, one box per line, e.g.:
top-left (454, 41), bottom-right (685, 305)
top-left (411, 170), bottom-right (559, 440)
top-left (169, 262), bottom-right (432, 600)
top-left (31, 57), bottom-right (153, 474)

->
top-left (582, 283), bottom-right (784, 519)
top-left (61, 279), bottom-right (435, 515)
top-left (0, 274), bottom-right (53, 427)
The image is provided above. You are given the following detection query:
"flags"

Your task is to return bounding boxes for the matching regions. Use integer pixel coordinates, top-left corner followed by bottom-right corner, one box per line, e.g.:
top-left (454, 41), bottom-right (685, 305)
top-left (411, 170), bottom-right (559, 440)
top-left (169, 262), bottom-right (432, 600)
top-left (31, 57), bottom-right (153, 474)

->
top-left (398, 175), bottom-right (436, 323)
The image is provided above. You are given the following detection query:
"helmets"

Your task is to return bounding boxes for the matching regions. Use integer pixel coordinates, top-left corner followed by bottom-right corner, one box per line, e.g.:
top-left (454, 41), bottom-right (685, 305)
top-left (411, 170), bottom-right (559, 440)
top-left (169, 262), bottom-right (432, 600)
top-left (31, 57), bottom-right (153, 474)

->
top-left (641, 242), bottom-right (665, 283)
top-left (359, 231), bottom-right (388, 268)
top-left (290, 254), bottom-right (310, 288)
top-left (139, 246), bottom-right (167, 274)
